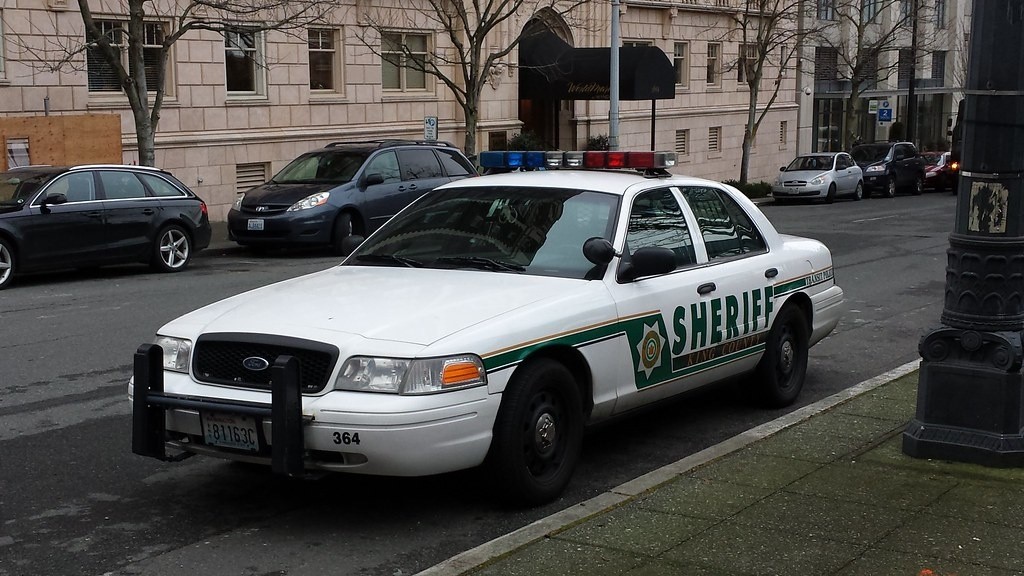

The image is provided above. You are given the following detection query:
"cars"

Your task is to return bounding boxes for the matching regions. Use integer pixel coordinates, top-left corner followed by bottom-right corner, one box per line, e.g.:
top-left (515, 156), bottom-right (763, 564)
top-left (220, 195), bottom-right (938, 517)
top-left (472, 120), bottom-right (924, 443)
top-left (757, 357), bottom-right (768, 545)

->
top-left (127, 151), bottom-right (845, 506)
top-left (918, 151), bottom-right (952, 192)
top-left (771, 152), bottom-right (864, 204)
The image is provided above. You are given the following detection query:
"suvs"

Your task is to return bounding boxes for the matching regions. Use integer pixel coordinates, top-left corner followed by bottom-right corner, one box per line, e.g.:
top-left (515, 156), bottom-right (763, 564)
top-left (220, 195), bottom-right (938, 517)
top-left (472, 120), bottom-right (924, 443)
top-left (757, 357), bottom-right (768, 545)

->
top-left (850, 140), bottom-right (926, 198)
top-left (227, 140), bottom-right (481, 256)
top-left (0, 164), bottom-right (212, 290)
